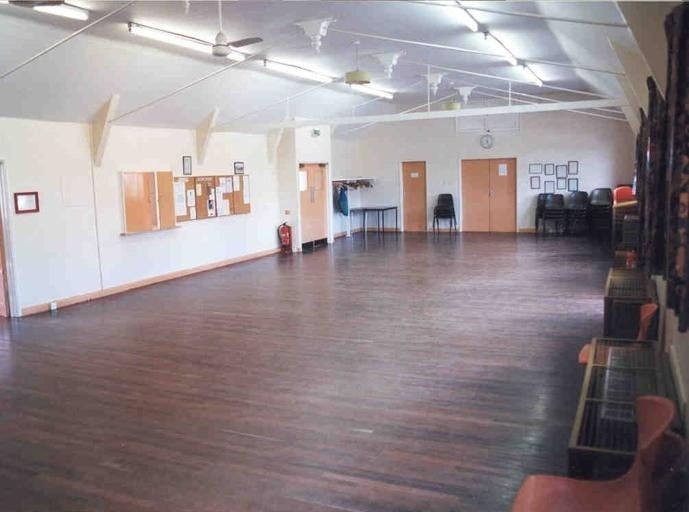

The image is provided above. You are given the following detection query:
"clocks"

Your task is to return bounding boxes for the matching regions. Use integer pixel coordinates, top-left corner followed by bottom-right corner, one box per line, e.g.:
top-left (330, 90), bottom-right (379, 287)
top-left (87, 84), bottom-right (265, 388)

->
top-left (480, 133), bottom-right (495, 150)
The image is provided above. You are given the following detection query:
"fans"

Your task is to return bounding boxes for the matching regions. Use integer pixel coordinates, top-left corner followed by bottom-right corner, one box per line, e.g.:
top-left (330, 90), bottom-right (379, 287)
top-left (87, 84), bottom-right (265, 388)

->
top-left (320, 39), bottom-right (400, 94)
top-left (178, 0), bottom-right (266, 62)
top-left (436, 82), bottom-right (474, 112)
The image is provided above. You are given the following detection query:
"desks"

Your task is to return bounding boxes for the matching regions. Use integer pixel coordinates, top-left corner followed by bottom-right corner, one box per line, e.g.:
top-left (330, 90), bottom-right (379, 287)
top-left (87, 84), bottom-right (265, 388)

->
top-left (565, 332), bottom-right (670, 478)
top-left (351, 205), bottom-right (400, 235)
top-left (602, 267), bottom-right (657, 336)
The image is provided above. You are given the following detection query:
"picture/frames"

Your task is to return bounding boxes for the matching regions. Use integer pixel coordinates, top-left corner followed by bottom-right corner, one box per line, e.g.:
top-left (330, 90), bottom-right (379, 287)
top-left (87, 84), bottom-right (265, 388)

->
top-left (528, 160), bottom-right (582, 194)
top-left (182, 156), bottom-right (191, 176)
top-left (232, 162), bottom-right (245, 175)
top-left (14, 190), bottom-right (42, 215)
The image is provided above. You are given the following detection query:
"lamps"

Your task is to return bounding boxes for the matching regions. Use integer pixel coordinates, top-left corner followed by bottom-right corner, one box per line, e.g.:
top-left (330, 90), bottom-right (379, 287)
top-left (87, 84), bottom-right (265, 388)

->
top-left (517, 60), bottom-right (544, 90)
top-left (120, 20), bottom-right (244, 63)
top-left (255, 57), bottom-right (332, 88)
top-left (447, 1), bottom-right (480, 35)
top-left (35, 1), bottom-right (90, 26)
top-left (331, 77), bottom-right (397, 102)
top-left (481, 33), bottom-right (519, 68)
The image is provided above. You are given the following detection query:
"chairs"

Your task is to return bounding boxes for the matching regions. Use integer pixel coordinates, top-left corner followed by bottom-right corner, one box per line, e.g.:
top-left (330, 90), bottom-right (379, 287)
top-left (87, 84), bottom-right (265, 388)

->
top-left (432, 193), bottom-right (457, 235)
top-left (528, 186), bottom-right (638, 269)
top-left (575, 304), bottom-right (664, 376)
top-left (511, 390), bottom-right (688, 512)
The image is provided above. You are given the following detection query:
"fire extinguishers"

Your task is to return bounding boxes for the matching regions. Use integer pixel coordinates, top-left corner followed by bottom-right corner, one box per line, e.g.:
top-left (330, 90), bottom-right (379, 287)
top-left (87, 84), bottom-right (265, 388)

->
top-left (278, 221), bottom-right (292, 255)
